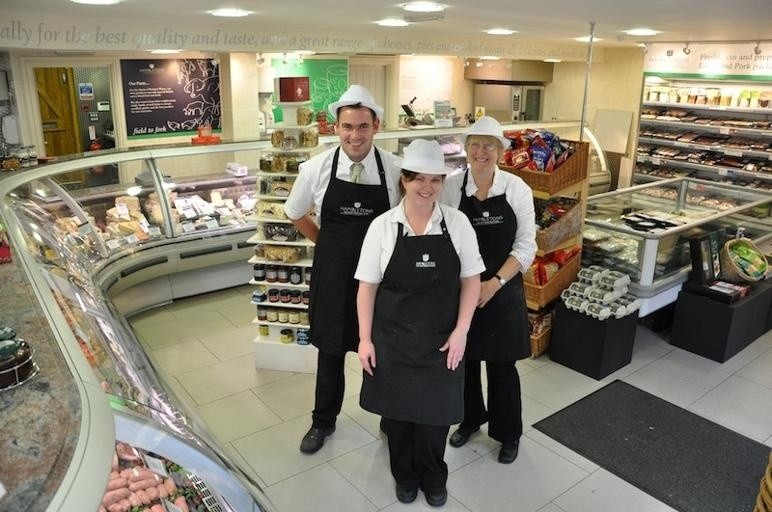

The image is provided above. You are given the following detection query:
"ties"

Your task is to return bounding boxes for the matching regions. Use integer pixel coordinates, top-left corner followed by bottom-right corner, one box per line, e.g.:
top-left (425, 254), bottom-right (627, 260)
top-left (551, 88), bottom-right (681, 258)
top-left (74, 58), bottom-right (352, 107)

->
top-left (352, 165), bottom-right (363, 184)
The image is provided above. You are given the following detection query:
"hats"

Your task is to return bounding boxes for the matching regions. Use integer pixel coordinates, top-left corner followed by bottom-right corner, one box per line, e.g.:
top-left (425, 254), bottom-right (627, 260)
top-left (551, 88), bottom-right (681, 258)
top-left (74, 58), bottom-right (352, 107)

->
top-left (328, 85), bottom-right (384, 119)
top-left (393, 139), bottom-right (454, 175)
top-left (457, 116), bottom-right (512, 150)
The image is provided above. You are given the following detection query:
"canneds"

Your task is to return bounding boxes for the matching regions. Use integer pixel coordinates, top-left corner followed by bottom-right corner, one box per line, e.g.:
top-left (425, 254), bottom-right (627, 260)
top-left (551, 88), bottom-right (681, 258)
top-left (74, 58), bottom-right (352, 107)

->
top-left (254, 262), bottom-right (312, 345)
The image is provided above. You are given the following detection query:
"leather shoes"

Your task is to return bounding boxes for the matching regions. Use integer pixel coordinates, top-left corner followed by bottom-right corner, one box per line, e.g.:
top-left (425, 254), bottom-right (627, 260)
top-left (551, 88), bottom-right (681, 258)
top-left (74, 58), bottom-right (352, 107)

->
top-left (499, 440), bottom-right (518, 463)
top-left (396, 483), bottom-right (418, 502)
top-left (425, 487), bottom-right (446, 505)
top-left (301, 426), bottom-right (335, 453)
top-left (450, 424), bottom-right (480, 446)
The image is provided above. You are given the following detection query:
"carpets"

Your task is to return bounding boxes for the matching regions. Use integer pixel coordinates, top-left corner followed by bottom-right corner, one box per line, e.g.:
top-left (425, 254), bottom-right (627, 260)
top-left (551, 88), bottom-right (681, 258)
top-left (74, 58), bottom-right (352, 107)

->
top-left (530, 379), bottom-right (772, 512)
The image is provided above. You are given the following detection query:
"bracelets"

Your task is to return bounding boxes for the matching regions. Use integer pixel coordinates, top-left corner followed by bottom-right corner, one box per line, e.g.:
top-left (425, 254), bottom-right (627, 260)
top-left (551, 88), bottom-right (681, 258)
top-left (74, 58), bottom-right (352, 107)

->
top-left (492, 274), bottom-right (507, 286)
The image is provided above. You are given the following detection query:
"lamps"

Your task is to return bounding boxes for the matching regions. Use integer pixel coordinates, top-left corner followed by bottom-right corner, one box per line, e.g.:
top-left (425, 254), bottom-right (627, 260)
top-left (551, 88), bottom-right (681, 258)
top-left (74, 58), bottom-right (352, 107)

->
top-left (619, 26), bottom-right (664, 37)
top-left (205, 7), bottom-right (255, 17)
top-left (479, 8), bottom-right (520, 34)
top-left (570, 35), bottom-right (603, 43)
top-left (370, 16), bottom-right (410, 27)
top-left (257, 52), bottom-right (276, 92)
top-left (683, 43), bottom-right (691, 54)
top-left (211, 57), bottom-right (220, 66)
top-left (396, 0), bottom-right (449, 13)
top-left (754, 40), bottom-right (762, 55)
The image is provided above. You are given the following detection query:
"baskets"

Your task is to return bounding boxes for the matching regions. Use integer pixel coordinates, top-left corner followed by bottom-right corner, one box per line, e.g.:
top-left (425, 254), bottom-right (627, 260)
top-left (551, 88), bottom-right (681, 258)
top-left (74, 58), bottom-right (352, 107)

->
top-left (524, 252), bottom-right (581, 307)
top-left (536, 196), bottom-right (581, 250)
top-left (530, 327), bottom-right (551, 357)
top-left (498, 139), bottom-right (589, 193)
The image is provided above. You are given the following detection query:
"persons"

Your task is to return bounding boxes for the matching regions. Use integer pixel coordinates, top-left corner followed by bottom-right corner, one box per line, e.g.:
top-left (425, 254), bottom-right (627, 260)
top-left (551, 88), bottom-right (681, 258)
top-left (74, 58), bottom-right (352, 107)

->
top-left (441, 114), bottom-right (539, 465)
top-left (281, 83), bottom-right (404, 455)
top-left (354, 135), bottom-right (488, 505)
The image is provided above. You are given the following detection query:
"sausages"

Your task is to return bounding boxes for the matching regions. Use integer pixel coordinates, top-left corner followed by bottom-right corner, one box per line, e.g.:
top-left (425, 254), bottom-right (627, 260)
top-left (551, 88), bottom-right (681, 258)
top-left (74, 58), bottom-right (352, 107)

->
top-left (96, 440), bottom-right (189, 512)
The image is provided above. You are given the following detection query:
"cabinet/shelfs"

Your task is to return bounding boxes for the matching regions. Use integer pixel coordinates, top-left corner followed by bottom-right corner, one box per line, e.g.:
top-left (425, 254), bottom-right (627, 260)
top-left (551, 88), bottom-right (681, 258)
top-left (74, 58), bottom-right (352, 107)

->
top-left (628, 71), bottom-right (772, 256)
top-left (244, 121), bottom-right (324, 374)
